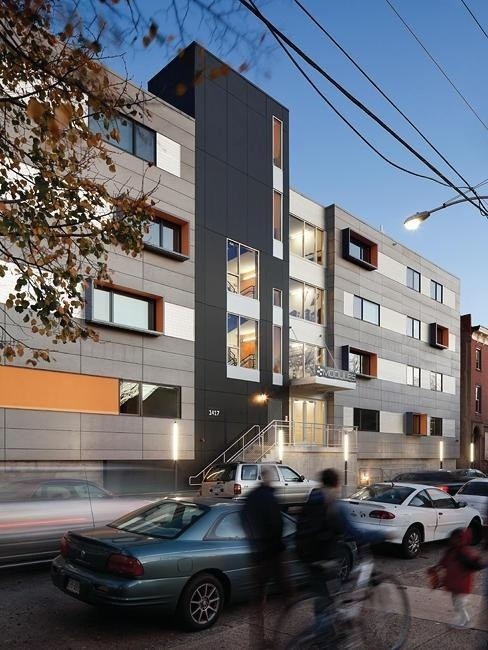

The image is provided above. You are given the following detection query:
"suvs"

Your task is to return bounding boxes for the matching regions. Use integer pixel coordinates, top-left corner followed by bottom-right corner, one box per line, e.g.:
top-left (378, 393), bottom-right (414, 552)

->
top-left (198, 461), bottom-right (328, 513)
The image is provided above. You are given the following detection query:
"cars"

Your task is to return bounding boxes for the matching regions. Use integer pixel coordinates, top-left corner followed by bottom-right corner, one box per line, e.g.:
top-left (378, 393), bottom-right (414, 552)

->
top-left (0, 477), bottom-right (169, 569)
top-left (344, 465), bottom-right (488, 559)
top-left (51, 496), bottom-right (363, 631)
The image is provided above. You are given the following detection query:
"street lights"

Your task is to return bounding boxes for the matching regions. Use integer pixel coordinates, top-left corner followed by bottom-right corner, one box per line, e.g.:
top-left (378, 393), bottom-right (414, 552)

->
top-left (403, 191), bottom-right (488, 235)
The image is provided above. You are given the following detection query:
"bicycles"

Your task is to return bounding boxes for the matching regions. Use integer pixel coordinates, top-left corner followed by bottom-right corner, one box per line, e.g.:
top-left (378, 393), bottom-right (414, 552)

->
top-left (268, 529), bottom-right (413, 649)
